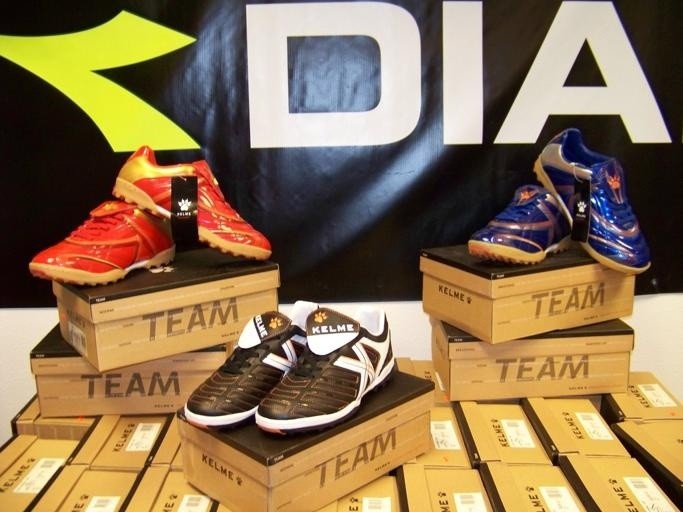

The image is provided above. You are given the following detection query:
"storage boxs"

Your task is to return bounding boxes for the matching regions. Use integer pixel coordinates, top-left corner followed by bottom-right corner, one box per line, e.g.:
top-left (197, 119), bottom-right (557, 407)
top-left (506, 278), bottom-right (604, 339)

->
top-left (28, 322), bottom-right (231, 418)
top-left (51, 246), bottom-right (282, 373)
top-left (418, 242), bottom-right (637, 345)
top-left (430, 316), bottom-right (633, 402)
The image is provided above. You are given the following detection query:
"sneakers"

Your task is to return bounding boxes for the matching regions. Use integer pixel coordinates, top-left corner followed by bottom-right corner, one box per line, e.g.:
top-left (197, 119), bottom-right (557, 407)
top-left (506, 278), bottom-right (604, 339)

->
top-left (185, 300), bottom-right (319, 430)
top-left (533, 129), bottom-right (651, 275)
top-left (255, 308), bottom-right (399, 436)
top-left (29, 202), bottom-right (176, 287)
top-left (112, 146), bottom-right (272, 261)
top-left (468, 185), bottom-right (577, 265)
